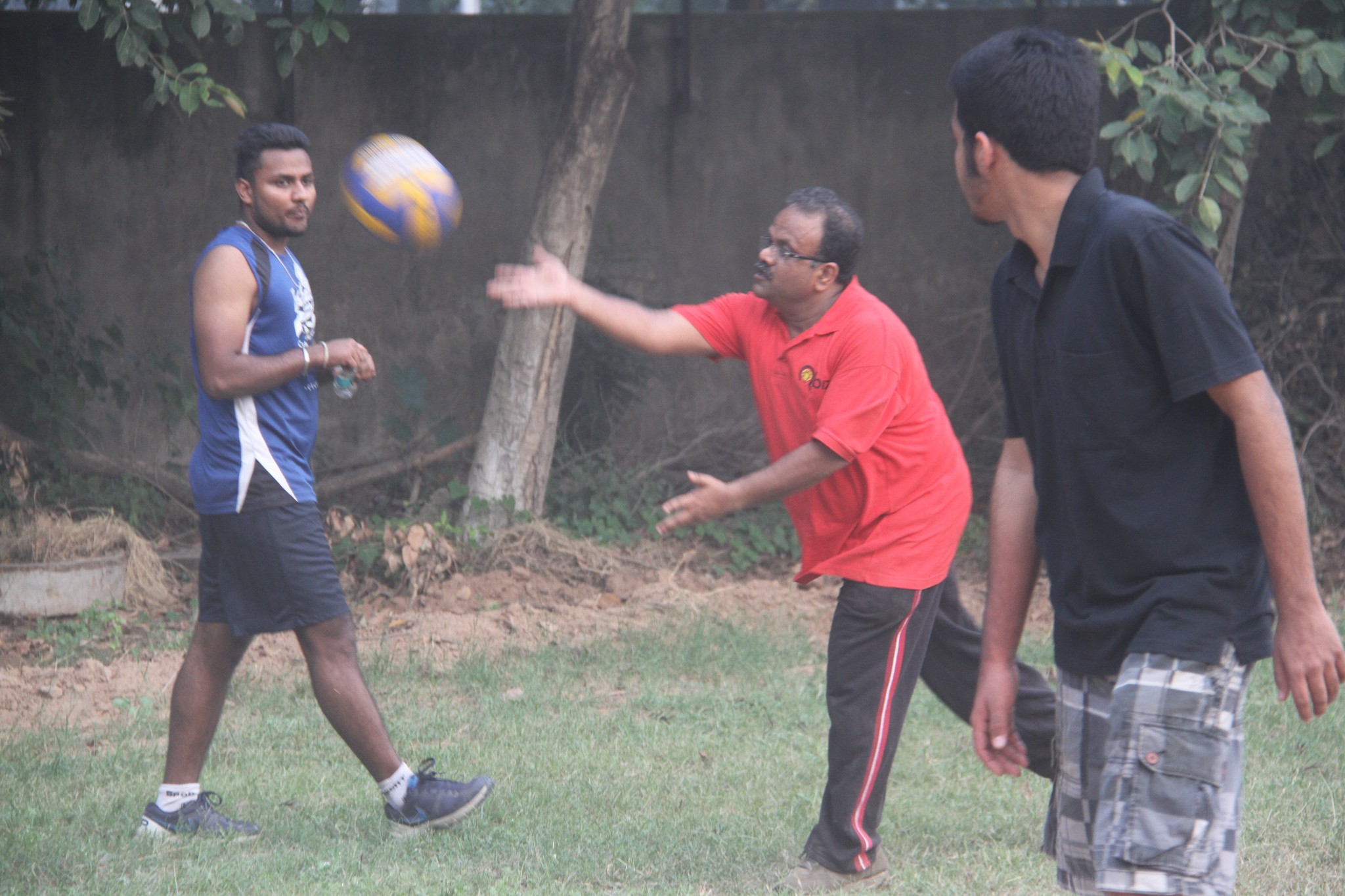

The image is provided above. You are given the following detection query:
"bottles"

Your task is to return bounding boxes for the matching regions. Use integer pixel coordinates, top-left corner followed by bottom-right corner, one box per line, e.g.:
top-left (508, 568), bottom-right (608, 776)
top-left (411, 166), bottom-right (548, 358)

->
top-left (331, 350), bottom-right (369, 400)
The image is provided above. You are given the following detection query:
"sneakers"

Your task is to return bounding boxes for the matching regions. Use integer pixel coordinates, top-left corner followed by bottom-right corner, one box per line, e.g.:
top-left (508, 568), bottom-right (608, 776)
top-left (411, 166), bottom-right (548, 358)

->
top-left (380, 757), bottom-right (490, 836)
top-left (137, 791), bottom-right (260, 846)
top-left (775, 847), bottom-right (888, 896)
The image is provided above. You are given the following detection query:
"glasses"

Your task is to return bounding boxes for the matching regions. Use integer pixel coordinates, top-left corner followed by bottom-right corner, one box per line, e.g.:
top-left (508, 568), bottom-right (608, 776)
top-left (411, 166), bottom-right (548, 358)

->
top-left (761, 235), bottom-right (826, 267)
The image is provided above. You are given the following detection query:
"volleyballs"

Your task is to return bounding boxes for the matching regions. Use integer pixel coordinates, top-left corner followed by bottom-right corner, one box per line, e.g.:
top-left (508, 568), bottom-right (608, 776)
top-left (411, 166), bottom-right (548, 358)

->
top-left (338, 135), bottom-right (463, 244)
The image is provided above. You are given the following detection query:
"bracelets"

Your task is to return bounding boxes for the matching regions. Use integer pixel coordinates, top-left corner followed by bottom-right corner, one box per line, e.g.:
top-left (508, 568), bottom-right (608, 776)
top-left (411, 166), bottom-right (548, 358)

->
top-left (301, 345), bottom-right (310, 379)
top-left (317, 340), bottom-right (330, 371)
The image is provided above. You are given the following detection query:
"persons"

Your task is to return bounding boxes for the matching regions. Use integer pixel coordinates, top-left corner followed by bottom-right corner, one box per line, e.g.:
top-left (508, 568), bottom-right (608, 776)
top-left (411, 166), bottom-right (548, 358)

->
top-left (136, 120), bottom-right (494, 842)
top-left (485, 185), bottom-right (1058, 896)
top-left (948, 29), bottom-right (1345, 896)
